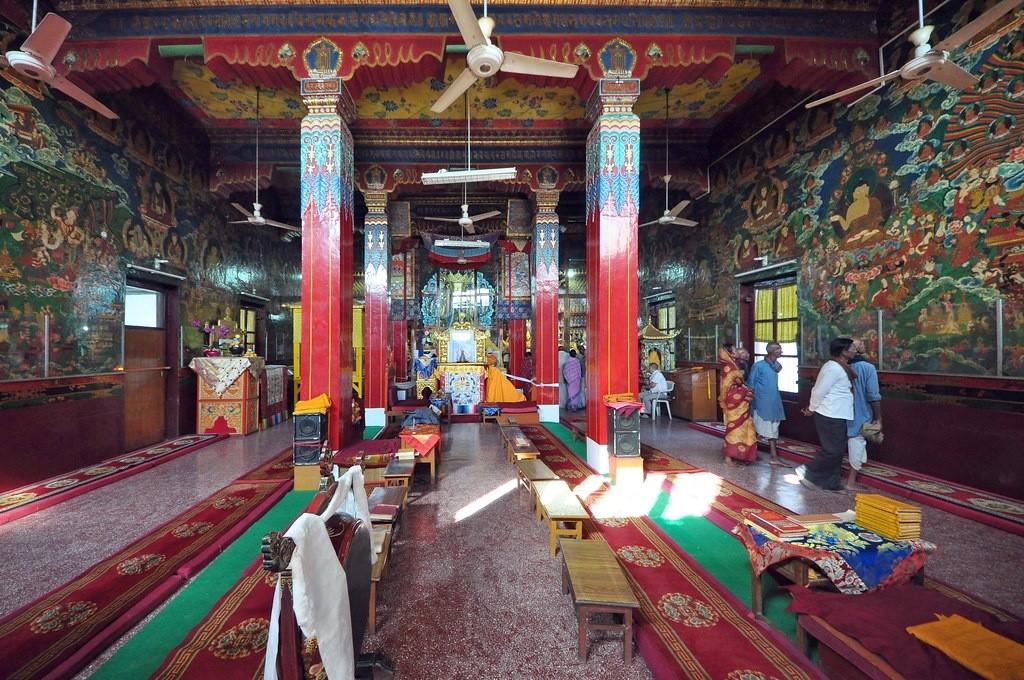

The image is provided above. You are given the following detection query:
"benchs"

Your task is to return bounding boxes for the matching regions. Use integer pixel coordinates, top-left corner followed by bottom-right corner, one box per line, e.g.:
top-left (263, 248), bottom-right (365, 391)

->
top-left (516, 459), bottom-right (560, 510)
top-left (558, 539), bottom-right (640, 663)
top-left (382, 459), bottom-right (418, 508)
top-left (500, 426), bottom-right (527, 458)
top-left (367, 487), bottom-right (409, 529)
top-left (496, 416), bottom-right (518, 435)
top-left (532, 480), bottom-right (590, 557)
top-left (509, 439), bottom-right (540, 466)
top-left (369, 524), bottom-right (392, 635)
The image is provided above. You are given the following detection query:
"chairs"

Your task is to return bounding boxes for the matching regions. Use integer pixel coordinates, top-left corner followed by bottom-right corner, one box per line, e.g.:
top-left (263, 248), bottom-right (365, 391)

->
top-left (641, 381), bottom-right (675, 421)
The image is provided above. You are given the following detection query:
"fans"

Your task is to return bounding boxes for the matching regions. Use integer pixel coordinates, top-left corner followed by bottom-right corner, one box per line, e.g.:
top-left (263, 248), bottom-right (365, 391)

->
top-left (804, 0), bottom-right (1024, 108)
top-left (227, 86), bottom-right (302, 233)
top-left (638, 87), bottom-right (699, 227)
top-left (0, 0), bottom-right (119, 120)
top-left (424, 89), bottom-right (500, 234)
top-left (428, 0), bottom-right (579, 114)
top-left (441, 248), bottom-right (475, 264)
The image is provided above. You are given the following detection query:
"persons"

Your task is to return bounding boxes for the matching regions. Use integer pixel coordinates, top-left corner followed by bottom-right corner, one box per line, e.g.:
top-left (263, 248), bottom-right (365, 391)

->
top-left (520, 346), bottom-right (587, 411)
top-left (638, 337), bottom-right (882, 494)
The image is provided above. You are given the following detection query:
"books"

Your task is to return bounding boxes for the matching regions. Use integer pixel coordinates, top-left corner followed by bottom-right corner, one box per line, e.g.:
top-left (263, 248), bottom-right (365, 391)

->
top-left (743, 494), bottom-right (922, 543)
top-left (513, 437), bottom-right (531, 447)
top-left (370, 504), bottom-right (400, 553)
top-left (398, 448), bottom-right (415, 461)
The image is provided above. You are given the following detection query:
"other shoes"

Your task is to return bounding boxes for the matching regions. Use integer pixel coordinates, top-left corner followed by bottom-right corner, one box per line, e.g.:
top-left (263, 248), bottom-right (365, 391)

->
top-left (643, 415), bottom-right (650, 419)
top-left (822, 485), bottom-right (849, 495)
top-left (641, 413), bottom-right (646, 418)
top-left (795, 463), bottom-right (816, 492)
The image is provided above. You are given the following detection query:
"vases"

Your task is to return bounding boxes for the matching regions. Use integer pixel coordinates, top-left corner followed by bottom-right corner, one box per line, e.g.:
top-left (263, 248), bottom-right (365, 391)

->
top-left (207, 349), bottom-right (221, 357)
top-left (229, 346), bottom-right (244, 356)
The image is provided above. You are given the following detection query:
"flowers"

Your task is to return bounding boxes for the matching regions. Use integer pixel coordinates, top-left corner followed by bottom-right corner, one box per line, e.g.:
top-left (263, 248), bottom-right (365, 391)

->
top-left (191, 320), bottom-right (230, 351)
top-left (230, 334), bottom-right (243, 348)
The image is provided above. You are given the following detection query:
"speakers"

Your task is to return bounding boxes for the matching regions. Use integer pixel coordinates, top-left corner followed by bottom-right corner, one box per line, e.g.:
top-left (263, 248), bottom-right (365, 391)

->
top-left (607, 407), bottom-right (640, 457)
top-left (293, 414), bottom-right (328, 465)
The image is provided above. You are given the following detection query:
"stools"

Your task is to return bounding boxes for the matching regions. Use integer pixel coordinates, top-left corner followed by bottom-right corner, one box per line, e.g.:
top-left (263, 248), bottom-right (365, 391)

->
top-left (480, 402), bottom-right (500, 424)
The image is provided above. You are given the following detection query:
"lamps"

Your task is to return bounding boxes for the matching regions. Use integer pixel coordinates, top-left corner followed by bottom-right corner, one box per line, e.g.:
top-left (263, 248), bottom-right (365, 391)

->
top-left (434, 183), bottom-right (490, 248)
top-left (422, 89), bottom-right (517, 185)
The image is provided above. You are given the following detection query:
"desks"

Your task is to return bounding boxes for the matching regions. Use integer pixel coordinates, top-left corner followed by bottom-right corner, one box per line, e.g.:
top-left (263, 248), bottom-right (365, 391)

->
top-left (731, 510), bottom-right (938, 659)
top-left (798, 583), bottom-right (1024, 680)
top-left (399, 424), bottom-right (441, 485)
top-left (430, 393), bottom-right (451, 424)
